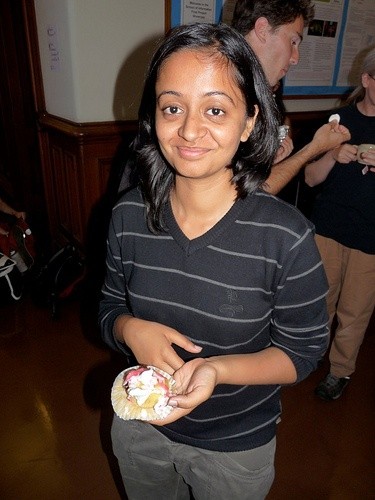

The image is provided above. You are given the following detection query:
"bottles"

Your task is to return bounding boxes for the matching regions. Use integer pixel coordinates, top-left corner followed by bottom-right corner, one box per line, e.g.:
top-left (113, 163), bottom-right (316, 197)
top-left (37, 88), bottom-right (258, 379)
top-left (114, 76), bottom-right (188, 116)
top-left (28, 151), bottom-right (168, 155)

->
top-left (10, 250), bottom-right (28, 272)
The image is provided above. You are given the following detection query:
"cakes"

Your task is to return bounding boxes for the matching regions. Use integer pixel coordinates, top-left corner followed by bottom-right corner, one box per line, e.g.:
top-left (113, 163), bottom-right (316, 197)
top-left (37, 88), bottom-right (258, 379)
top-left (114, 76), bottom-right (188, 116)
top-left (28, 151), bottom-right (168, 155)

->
top-left (123, 364), bottom-right (172, 413)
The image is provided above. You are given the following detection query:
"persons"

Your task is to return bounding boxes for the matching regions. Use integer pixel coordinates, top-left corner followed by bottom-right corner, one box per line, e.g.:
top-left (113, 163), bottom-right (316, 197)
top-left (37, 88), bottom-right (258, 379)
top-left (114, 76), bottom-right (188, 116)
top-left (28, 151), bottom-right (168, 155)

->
top-left (0, 199), bottom-right (25, 237)
top-left (230, 1), bottom-right (351, 196)
top-left (100, 24), bottom-right (330, 500)
top-left (294, 45), bottom-right (375, 403)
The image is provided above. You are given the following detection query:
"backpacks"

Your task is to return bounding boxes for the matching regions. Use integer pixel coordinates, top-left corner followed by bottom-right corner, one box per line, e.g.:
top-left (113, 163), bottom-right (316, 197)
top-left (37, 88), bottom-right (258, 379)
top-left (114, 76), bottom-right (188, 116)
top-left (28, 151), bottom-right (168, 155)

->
top-left (29, 233), bottom-right (88, 320)
top-left (0, 217), bottom-right (36, 305)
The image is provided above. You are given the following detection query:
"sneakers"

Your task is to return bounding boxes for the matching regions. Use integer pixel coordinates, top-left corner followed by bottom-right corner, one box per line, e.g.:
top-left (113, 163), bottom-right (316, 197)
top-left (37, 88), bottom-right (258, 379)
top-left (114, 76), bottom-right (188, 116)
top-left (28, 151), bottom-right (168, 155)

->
top-left (314, 372), bottom-right (350, 400)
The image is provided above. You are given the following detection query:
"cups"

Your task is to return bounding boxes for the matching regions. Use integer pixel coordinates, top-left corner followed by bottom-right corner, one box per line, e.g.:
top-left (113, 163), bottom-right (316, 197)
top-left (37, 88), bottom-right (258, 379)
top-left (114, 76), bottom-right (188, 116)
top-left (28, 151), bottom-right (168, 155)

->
top-left (278, 125), bottom-right (289, 145)
top-left (358, 144), bottom-right (375, 165)
